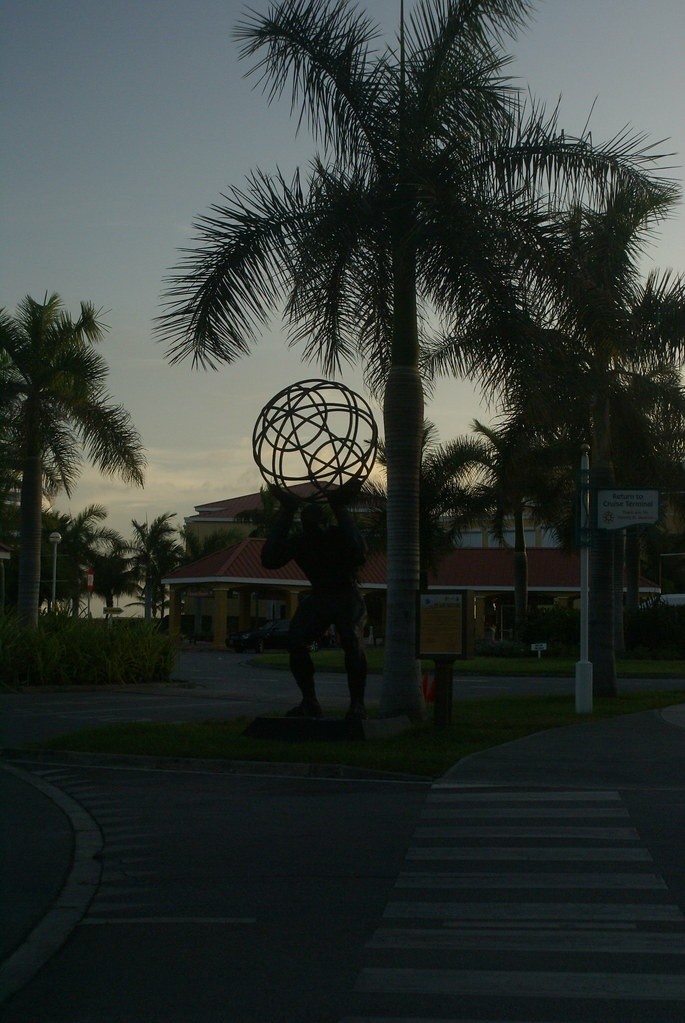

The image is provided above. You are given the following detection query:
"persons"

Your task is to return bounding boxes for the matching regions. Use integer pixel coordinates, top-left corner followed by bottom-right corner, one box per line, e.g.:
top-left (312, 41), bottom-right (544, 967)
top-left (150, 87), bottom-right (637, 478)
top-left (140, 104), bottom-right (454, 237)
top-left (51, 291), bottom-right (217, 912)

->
top-left (260, 479), bottom-right (372, 721)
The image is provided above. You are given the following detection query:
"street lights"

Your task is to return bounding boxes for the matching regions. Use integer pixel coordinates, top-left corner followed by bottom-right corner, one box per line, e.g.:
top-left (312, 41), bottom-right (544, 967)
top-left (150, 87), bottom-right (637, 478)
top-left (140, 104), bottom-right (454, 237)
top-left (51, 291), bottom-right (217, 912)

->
top-left (49, 531), bottom-right (63, 614)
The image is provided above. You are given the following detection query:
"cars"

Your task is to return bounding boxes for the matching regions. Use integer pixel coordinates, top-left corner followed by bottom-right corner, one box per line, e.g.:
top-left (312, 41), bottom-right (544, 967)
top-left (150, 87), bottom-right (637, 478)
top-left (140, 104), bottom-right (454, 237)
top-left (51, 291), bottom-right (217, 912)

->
top-left (225, 619), bottom-right (320, 654)
